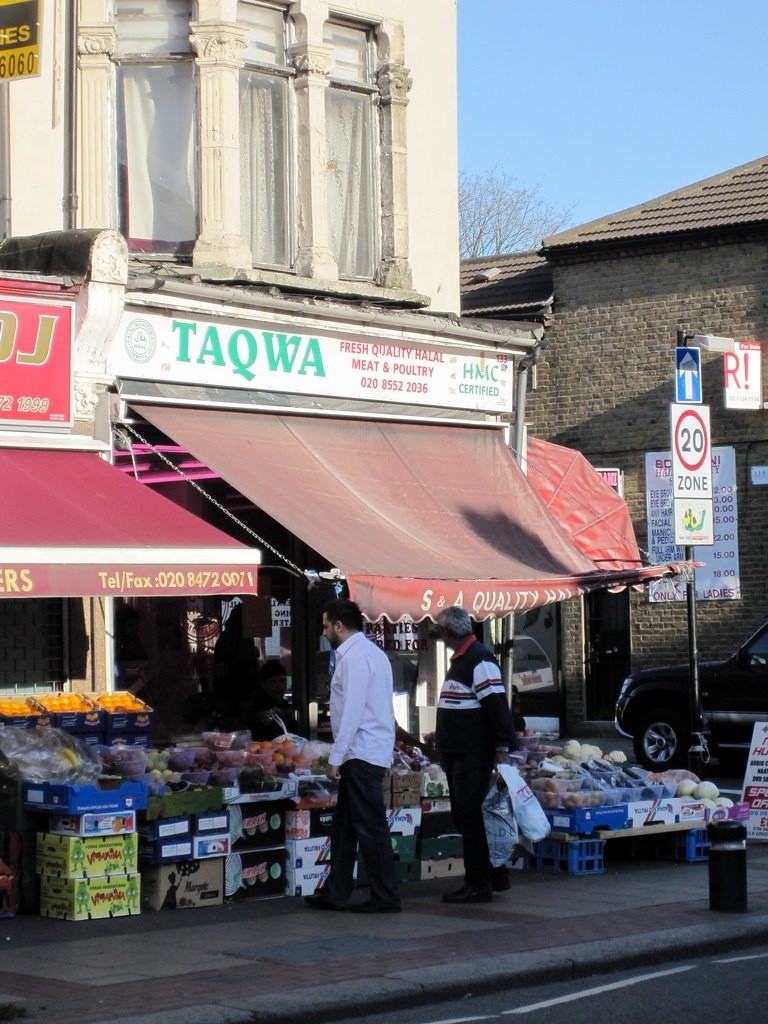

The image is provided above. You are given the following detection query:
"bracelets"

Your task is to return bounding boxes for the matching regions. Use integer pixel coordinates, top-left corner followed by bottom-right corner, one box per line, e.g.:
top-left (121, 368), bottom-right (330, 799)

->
top-left (495, 746), bottom-right (508, 752)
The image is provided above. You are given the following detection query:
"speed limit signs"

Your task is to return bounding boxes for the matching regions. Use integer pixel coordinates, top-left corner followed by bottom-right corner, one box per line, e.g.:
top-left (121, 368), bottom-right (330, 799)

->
top-left (667, 402), bottom-right (712, 498)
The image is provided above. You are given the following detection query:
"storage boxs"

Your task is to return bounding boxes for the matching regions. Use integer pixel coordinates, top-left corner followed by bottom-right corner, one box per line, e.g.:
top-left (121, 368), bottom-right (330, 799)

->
top-left (0, 691), bottom-right (746, 922)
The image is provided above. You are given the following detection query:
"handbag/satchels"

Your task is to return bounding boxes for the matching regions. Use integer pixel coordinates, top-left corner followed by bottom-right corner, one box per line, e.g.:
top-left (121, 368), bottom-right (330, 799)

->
top-left (481, 773), bottom-right (535, 869)
top-left (497, 764), bottom-right (551, 843)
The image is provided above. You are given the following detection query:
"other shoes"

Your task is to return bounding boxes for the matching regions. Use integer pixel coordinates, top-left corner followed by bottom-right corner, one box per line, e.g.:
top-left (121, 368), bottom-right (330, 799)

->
top-left (304, 895), bottom-right (347, 911)
top-left (349, 900), bottom-right (401, 914)
top-left (492, 877), bottom-right (510, 892)
top-left (442, 883), bottom-right (492, 904)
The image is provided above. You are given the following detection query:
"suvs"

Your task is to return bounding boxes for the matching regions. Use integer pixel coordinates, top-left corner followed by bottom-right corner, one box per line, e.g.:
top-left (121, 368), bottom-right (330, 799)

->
top-left (612, 620), bottom-right (768, 770)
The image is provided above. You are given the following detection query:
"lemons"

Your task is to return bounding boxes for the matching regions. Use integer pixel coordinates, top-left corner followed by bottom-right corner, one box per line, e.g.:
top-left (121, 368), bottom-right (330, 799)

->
top-left (142, 750), bottom-right (179, 786)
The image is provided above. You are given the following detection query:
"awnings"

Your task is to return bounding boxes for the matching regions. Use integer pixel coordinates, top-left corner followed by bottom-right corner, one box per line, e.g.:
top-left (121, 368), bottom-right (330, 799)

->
top-left (0, 429), bottom-right (262, 599)
top-left (129, 402), bottom-right (705, 623)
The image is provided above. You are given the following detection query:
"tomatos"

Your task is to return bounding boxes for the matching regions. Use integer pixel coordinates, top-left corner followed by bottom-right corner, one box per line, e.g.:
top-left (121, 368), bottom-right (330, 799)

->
top-left (247, 739), bottom-right (308, 764)
top-left (512, 729), bottom-right (604, 808)
top-left (0, 694), bottom-right (144, 717)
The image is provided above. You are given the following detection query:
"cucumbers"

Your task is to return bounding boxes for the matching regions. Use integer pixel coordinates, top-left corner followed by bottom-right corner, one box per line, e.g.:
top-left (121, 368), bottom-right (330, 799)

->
top-left (581, 760), bottom-right (641, 779)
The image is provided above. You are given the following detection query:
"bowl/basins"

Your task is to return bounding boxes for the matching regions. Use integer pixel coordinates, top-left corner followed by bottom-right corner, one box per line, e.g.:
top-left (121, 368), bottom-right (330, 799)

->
top-left (114, 760), bottom-right (147, 775)
top-left (202, 731), bottom-right (234, 748)
top-left (536, 790), bottom-right (608, 809)
top-left (152, 773), bottom-right (183, 785)
top-left (93, 744), bottom-right (115, 773)
top-left (179, 771), bottom-right (210, 786)
top-left (209, 769), bottom-right (238, 786)
top-left (538, 745), bottom-right (563, 757)
top-left (601, 786), bottom-right (645, 805)
top-left (590, 766), bottom-right (623, 785)
top-left (244, 751), bottom-right (275, 763)
top-left (644, 784), bottom-right (679, 800)
top-left (165, 747), bottom-right (246, 768)
top-left (230, 729), bottom-right (252, 747)
top-left (627, 768), bottom-right (649, 784)
top-left (528, 751), bottom-right (549, 767)
top-left (552, 774), bottom-right (585, 792)
top-left (109, 744), bottom-right (144, 754)
top-left (513, 734), bottom-right (539, 749)
top-left (245, 780), bottom-right (283, 791)
top-left (496, 750), bottom-right (529, 764)
top-left (131, 773), bottom-right (156, 783)
top-left (276, 761), bottom-right (313, 774)
top-left (519, 773), bottom-right (527, 778)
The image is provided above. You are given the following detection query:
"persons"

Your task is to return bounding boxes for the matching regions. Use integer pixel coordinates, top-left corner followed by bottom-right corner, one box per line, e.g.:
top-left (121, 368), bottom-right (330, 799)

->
top-left (213, 603), bottom-right (260, 728)
top-left (116, 607), bottom-right (150, 699)
top-left (435, 606), bottom-right (517, 904)
top-left (304, 599), bottom-right (402, 912)
top-left (247, 662), bottom-right (297, 741)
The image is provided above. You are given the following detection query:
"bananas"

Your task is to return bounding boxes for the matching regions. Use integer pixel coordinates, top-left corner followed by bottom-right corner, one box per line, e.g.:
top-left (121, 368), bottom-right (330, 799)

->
top-left (59, 748), bottom-right (78, 766)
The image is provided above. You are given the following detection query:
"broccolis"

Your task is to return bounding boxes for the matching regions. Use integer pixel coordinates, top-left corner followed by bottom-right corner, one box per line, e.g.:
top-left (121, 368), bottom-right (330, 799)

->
top-left (550, 739), bottom-right (627, 766)
